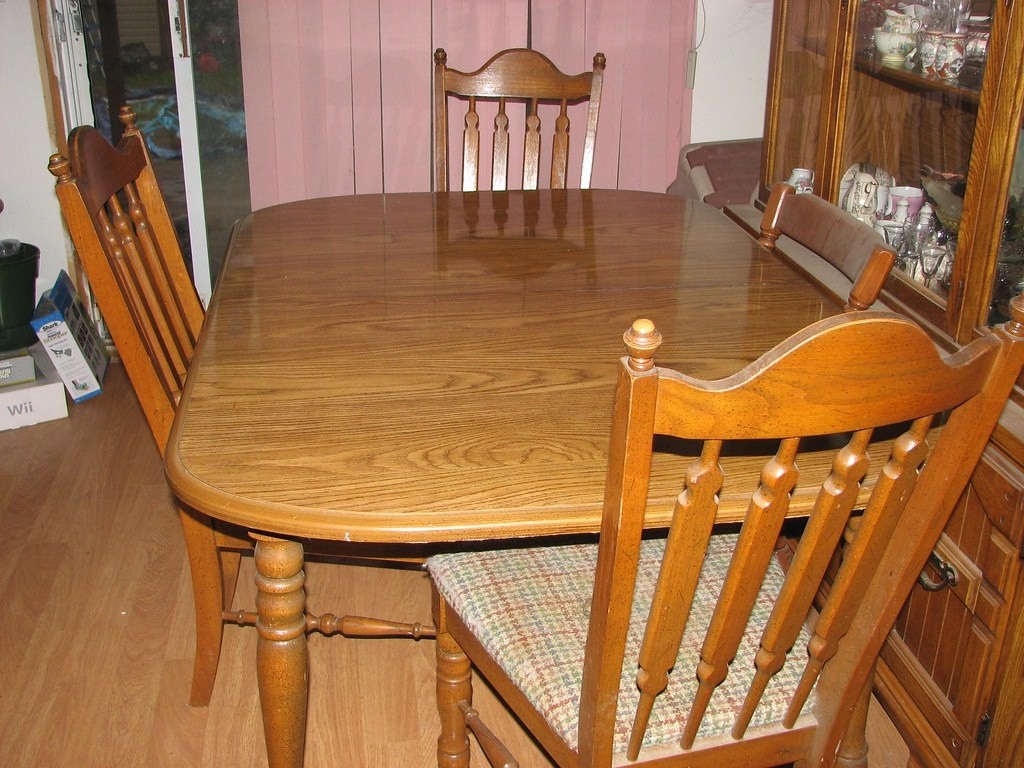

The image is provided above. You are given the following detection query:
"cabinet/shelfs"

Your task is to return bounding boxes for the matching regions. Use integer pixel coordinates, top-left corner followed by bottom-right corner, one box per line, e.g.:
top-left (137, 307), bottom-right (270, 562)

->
top-left (753, 0), bottom-right (1024, 412)
top-left (954, 1), bottom-right (1024, 411)
top-left (722, 203), bottom-right (1024, 768)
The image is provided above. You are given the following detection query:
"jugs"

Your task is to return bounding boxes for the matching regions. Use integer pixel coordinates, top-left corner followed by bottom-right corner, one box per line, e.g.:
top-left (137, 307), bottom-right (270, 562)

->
top-left (880, 9), bottom-right (922, 35)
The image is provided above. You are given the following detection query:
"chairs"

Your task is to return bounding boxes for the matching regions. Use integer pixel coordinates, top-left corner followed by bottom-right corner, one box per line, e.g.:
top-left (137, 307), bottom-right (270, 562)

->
top-left (429, 47), bottom-right (608, 189)
top-left (421, 287), bottom-right (1024, 768)
top-left (47, 104), bottom-right (446, 709)
top-left (756, 182), bottom-right (897, 315)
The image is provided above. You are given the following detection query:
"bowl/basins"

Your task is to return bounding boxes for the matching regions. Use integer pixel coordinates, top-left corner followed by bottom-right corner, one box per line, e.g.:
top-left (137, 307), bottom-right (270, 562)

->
top-left (870, 24), bottom-right (918, 60)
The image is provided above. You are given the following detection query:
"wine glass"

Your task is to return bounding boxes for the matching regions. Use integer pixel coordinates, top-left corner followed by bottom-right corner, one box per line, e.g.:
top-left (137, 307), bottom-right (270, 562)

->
top-left (883, 217), bottom-right (945, 293)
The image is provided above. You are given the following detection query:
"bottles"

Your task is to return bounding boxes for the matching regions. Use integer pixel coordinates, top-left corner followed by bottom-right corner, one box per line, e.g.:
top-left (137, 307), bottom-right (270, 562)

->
top-left (890, 198), bottom-right (933, 225)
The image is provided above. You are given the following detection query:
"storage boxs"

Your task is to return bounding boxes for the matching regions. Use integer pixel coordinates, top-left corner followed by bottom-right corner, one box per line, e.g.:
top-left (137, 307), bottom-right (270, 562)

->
top-left (29, 269), bottom-right (111, 403)
top-left (0, 378), bottom-right (72, 432)
top-left (0, 347), bottom-right (37, 388)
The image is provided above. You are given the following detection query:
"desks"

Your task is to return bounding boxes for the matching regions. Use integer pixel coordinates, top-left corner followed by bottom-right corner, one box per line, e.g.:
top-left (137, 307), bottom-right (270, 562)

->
top-left (162, 191), bottom-right (947, 768)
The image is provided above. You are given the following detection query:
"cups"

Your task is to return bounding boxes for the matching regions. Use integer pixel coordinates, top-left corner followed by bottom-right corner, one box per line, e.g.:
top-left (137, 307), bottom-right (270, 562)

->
top-left (943, 236), bottom-right (960, 288)
top-left (913, 0), bottom-right (972, 32)
top-left (918, 29), bottom-right (967, 81)
top-left (875, 219), bottom-right (895, 244)
top-left (785, 167), bottom-right (815, 193)
top-left (885, 185), bottom-right (924, 222)
